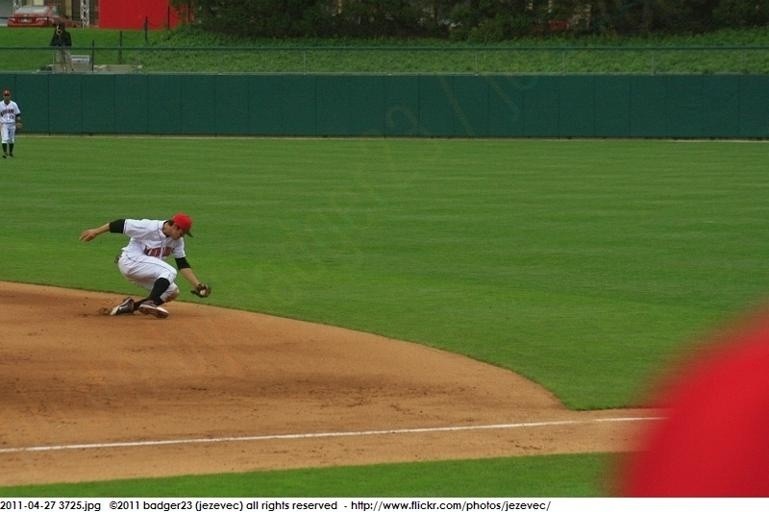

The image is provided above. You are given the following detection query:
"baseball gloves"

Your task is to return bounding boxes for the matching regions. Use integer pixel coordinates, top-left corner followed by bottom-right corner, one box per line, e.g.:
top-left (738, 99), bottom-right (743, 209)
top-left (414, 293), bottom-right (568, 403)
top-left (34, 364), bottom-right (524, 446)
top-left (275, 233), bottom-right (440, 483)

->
top-left (16, 122), bottom-right (23, 130)
top-left (191, 284), bottom-right (211, 297)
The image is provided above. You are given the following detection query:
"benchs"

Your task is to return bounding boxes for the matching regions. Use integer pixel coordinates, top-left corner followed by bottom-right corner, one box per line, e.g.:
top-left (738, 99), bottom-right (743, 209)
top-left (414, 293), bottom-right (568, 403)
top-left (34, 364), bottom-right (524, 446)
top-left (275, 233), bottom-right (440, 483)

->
top-left (68, 53), bottom-right (91, 72)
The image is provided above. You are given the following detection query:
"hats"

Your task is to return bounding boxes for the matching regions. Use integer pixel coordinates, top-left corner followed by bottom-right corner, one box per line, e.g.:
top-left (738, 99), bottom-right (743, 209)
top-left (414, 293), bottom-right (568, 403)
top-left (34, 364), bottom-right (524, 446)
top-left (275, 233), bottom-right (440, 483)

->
top-left (169, 213), bottom-right (194, 238)
top-left (3, 90), bottom-right (10, 97)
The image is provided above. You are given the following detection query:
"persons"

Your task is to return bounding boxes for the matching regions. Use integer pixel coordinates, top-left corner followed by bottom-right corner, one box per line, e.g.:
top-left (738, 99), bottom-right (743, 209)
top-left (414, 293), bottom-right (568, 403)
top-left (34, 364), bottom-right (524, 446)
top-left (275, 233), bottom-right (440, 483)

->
top-left (49, 23), bottom-right (74, 71)
top-left (0, 89), bottom-right (23, 160)
top-left (76, 211), bottom-right (211, 319)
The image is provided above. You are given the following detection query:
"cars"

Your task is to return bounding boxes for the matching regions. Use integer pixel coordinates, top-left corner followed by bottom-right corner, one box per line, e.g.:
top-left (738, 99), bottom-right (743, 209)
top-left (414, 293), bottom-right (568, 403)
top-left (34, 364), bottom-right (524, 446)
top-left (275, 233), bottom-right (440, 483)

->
top-left (8, 5), bottom-right (61, 28)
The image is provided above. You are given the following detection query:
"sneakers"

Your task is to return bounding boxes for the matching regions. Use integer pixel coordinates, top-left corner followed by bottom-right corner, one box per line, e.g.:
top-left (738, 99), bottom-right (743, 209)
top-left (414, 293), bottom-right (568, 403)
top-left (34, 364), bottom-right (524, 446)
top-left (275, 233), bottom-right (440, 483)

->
top-left (3, 152), bottom-right (9, 159)
top-left (137, 300), bottom-right (168, 319)
top-left (110, 296), bottom-right (135, 317)
